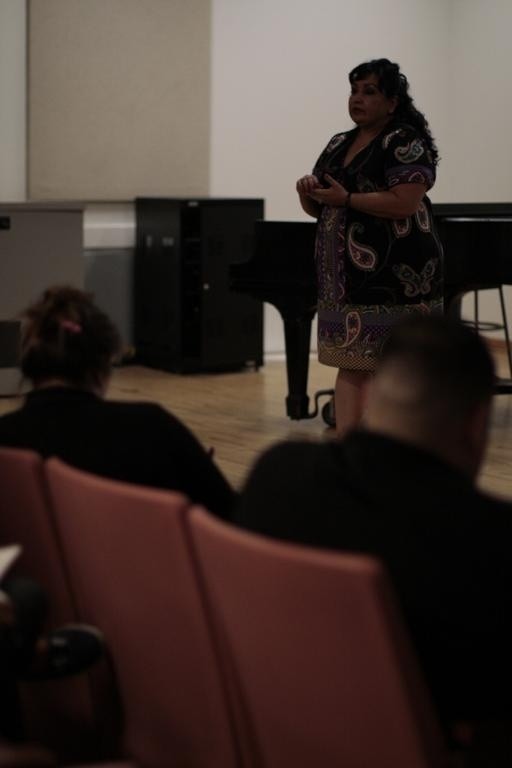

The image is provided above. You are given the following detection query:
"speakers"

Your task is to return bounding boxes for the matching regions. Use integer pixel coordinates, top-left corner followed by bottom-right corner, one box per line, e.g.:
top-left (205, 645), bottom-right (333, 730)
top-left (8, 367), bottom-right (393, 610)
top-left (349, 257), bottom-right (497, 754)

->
top-left (134, 193), bottom-right (266, 377)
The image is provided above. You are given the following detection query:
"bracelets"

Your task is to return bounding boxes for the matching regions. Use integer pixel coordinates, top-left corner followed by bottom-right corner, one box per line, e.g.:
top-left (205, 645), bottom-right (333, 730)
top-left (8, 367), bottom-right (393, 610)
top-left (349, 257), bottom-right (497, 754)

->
top-left (345, 192), bottom-right (352, 207)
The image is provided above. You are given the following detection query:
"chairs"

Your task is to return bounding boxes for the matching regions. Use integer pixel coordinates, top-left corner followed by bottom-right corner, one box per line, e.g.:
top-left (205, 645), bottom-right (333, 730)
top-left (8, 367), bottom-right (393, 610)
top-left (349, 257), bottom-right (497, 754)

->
top-left (1, 446), bottom-right (425, 766)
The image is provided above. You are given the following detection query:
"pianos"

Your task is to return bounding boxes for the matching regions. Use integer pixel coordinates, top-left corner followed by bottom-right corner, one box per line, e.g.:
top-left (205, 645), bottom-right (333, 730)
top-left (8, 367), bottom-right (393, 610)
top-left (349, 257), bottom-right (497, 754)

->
top-left (245, 204), bottom-right (510, 420)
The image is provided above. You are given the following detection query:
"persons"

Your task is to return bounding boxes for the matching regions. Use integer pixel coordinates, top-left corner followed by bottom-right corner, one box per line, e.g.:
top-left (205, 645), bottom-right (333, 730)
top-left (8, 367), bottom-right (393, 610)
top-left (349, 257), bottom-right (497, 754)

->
top-left (0, 284), bottom-right (243, 524)
top-left (244, 313), bottom-right (510, 765)
top-left (295, 55), bottom-right (450, 435)
